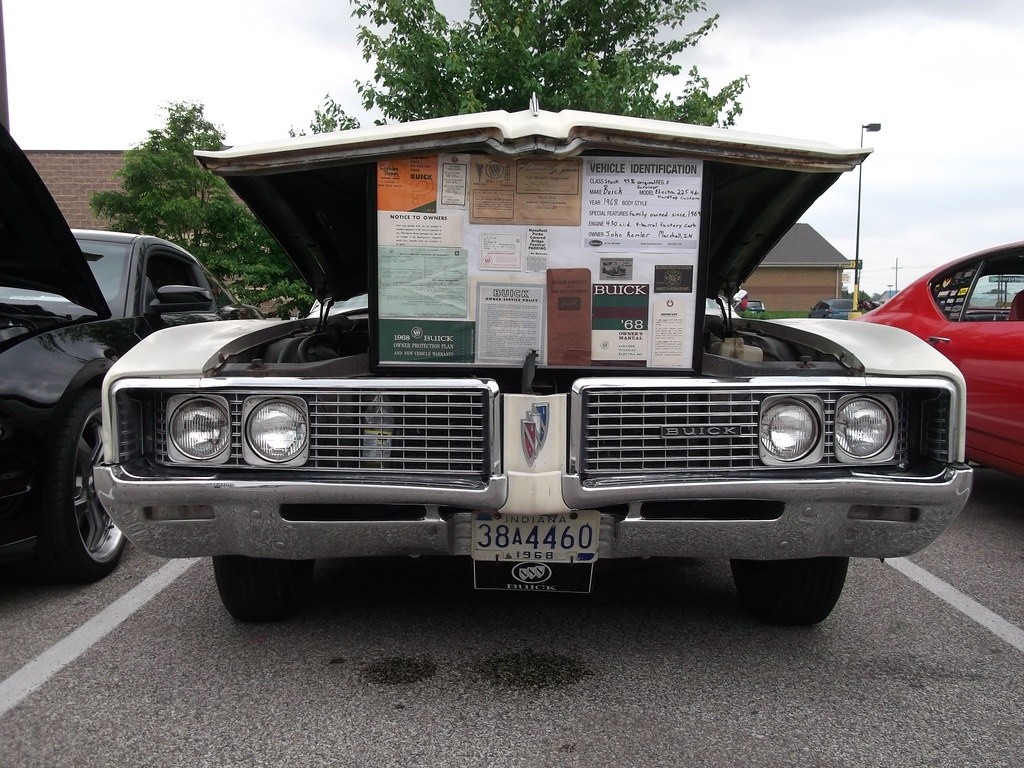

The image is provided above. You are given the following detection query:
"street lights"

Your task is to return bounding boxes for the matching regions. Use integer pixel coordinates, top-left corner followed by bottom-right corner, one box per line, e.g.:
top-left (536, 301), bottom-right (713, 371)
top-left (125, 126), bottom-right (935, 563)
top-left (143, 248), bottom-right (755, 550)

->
top-left (848, 120), bottom-right (882, 321)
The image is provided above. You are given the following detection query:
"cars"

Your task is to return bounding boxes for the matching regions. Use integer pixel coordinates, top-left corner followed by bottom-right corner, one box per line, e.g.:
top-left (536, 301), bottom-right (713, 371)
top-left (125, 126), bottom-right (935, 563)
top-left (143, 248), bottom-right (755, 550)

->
top-left (95, 96), bottom-right (968, 624)
top-left (808, 298), bottom-right (854, 320)
top-left (704, 296), bottom-right (742, 319)
top-left (740, 298), bottom-right (766, 313)
top-left (856, 242), bottom-right (1024, 475)
top-left (1, 124), bottom-right (265, 583)
